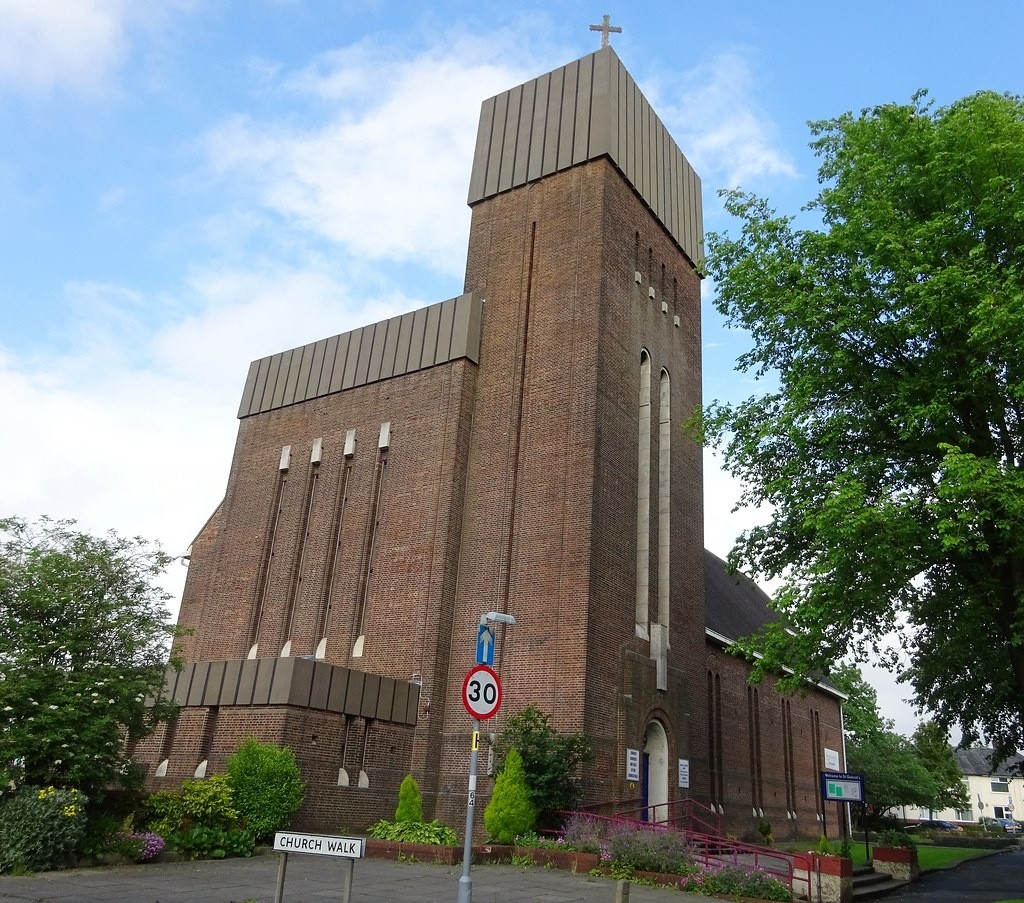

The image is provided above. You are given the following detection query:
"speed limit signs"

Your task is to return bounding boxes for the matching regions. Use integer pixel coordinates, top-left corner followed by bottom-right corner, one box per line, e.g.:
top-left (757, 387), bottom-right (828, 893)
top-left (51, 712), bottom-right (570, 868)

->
top-left (1008, 803), bottom-right (1014, 811)
top-left (462, 665), bottom-right (503, 719)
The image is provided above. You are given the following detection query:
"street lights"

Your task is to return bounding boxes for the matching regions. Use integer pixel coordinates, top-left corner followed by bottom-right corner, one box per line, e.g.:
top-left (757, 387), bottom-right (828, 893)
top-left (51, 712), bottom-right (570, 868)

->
top-left (453, 611), bottom-right (517, 903)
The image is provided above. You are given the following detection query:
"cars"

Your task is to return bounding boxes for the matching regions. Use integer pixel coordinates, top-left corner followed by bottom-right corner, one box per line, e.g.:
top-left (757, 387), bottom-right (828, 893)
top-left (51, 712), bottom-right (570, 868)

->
top-left (903, 820), bottom-right (960, 835)
top-left (980, 818), bottom-right (1023, 833)
